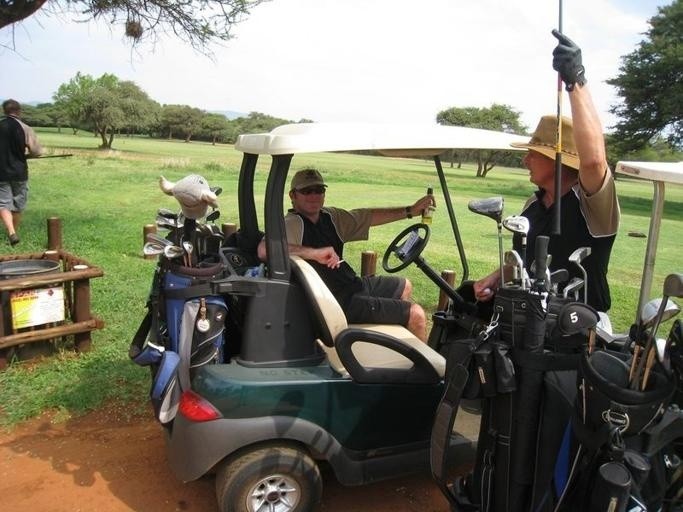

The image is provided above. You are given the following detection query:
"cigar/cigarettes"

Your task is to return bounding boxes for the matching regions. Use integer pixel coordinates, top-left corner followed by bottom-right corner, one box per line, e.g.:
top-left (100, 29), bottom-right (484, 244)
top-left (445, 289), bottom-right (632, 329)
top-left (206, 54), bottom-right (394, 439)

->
top-left (336, 259), bottom-right (345, 264)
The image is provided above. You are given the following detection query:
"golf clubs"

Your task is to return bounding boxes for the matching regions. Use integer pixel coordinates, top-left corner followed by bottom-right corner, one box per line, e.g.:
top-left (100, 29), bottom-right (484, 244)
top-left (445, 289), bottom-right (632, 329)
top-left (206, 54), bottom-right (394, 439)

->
top-left (625, 274), bottom-right (683, 392)
top-left (143, 187), bottom-right (226, 267)
top-left (467, 196), bottom-right (601, 357)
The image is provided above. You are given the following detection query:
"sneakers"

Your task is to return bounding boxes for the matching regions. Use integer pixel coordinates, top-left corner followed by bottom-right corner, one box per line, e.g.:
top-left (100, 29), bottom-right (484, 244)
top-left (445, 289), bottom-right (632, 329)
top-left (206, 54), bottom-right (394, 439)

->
top-left (8, 233), bottom-right (20, 246)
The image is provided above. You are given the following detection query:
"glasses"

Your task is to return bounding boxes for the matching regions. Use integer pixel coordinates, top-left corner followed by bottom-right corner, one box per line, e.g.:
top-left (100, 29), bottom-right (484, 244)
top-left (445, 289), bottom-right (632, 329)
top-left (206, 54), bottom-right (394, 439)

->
top-left (297, 186), bottom-right (326, 195)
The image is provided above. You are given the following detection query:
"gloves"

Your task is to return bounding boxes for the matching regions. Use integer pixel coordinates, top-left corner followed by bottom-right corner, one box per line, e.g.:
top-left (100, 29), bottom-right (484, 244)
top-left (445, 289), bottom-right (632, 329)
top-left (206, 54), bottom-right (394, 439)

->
top-left (550, 27), bottom-right (587, 93)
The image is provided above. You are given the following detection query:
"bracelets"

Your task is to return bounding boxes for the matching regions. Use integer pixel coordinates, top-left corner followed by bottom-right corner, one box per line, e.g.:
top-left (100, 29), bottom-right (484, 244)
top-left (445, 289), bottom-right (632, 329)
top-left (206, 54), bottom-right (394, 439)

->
top-left (404, 205), bottom-right (413, 220)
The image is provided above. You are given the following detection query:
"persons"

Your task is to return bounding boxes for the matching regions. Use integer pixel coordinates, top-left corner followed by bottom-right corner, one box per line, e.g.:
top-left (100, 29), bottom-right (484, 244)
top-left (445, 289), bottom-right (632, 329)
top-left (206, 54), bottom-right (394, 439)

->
top-left (471, 27), bottom-right (622, 346)
top-left (0, 98), bottom-right (42, 245)
top-left (256, 164), bottom-right (437, 344)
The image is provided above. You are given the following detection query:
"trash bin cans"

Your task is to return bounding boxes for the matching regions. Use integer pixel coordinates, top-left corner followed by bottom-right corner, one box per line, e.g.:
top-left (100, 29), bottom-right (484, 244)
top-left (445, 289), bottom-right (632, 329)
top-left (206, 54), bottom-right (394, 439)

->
top-left (0, 259), bottom-right (62, 364)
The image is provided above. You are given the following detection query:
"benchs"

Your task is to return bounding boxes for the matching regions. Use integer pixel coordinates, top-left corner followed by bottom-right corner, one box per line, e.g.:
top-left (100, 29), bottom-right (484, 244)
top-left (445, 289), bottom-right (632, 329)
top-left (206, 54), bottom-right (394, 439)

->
top-left (288, 251), bottom-right (449, 378)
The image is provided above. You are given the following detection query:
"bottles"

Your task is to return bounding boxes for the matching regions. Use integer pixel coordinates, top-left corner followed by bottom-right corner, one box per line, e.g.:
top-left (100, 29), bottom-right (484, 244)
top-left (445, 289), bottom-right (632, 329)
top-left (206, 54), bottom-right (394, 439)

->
top-left (244, 262), bottom-right (265, 278)
top-left (420, 187), bottom-right (433, 226)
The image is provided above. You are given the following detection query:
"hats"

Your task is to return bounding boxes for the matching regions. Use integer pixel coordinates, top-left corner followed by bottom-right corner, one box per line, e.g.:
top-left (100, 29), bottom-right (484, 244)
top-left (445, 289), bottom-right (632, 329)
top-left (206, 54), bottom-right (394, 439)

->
top-left (509, 113), bottom-right (581, 171)
top-left (290, 169), bottom-right (328, 192)
